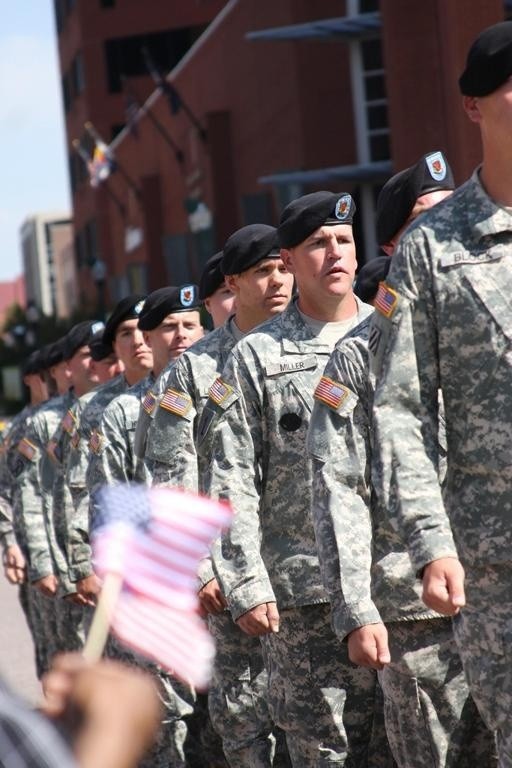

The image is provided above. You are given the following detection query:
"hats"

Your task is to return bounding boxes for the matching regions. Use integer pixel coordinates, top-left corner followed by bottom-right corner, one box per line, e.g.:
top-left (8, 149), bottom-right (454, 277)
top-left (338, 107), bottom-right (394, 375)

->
top-left (353, 256), bottom-right (392, 303)
top-left (376, 151), bottom-right (454, 246)
top-left (22, 191), bottom-right (356, 374)
top-left (459, 21), bottom-right (512, 96)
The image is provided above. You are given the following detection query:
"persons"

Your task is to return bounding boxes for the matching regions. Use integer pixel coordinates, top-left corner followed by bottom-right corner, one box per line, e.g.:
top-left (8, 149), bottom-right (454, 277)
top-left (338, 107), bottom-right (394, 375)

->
top-left (369, 15), bottom-right (512, 768)
top-left (307, 144), bottom-right (504, 768)
